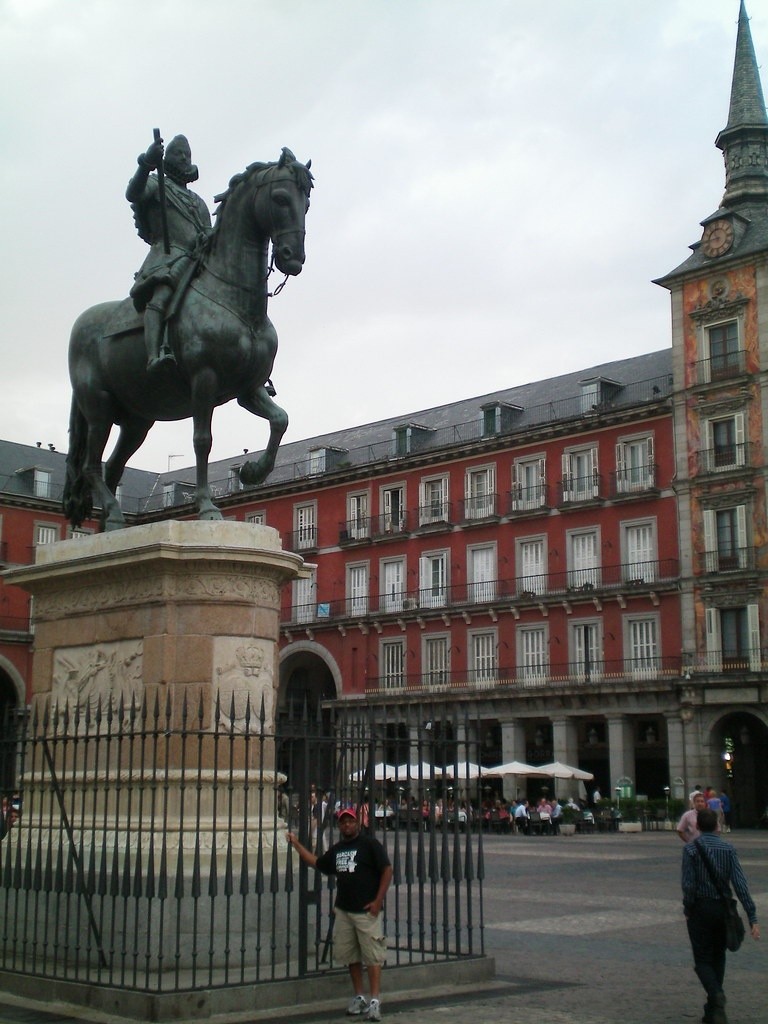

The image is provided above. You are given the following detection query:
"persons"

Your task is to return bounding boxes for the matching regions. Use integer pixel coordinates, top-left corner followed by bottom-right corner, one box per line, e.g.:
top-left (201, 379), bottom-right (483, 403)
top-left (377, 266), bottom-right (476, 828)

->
top-left (0, 790), bottom-right (21, 840)
top-left (677, 784), bottom-right (730, 843)
top-left (285, 808), bottom-right (393, 1021)
top-left (278, 784), bottom-right (602, 848)
top-left (125, 134), bottom-right (212, 374)
top-left (681, 808), bottom-right (761, 1024)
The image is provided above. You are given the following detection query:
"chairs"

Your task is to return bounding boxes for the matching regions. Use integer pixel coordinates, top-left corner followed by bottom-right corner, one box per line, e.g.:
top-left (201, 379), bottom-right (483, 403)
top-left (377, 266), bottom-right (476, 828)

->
top-left (182, 485), bottom-right (222, 504)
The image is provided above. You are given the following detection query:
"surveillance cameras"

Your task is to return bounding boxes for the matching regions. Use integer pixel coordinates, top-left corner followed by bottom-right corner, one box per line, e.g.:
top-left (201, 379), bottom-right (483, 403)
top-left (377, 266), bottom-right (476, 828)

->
top-left (686, 674), bottom-right (690, 681)
top-left (425, 722), bottom-right (432, 731)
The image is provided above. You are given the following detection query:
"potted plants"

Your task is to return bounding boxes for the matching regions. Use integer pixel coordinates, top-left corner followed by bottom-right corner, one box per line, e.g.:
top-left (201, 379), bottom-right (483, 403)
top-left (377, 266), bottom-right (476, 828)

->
top-left (559, 797), bottom-right (689, 836)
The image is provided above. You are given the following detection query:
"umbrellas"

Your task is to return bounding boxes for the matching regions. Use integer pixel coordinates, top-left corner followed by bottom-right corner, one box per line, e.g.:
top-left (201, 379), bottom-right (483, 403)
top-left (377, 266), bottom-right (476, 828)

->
top-left (349, 761), bottom-right (594, 802)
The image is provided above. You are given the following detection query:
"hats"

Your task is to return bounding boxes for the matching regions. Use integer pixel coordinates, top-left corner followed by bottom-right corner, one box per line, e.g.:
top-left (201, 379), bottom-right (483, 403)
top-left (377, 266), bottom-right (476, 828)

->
top-left (336, 809), bottom-right (357, 822)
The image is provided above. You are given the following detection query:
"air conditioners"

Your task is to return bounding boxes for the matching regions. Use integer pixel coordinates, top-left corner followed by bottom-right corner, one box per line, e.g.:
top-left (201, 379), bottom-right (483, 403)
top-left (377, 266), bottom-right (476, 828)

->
top-left (402, 597), bottom-right (417, 610)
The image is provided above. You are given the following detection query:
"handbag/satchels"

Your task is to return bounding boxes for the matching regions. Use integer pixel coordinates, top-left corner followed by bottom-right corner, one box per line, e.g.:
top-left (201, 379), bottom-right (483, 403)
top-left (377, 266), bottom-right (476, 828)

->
top-left (723, 898), bottom-right (745, 952)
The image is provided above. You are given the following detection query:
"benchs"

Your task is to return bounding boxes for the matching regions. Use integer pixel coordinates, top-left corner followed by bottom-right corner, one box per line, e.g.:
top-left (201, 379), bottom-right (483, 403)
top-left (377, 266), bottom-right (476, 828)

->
top-left (396, 808), bottom-right (559, 835)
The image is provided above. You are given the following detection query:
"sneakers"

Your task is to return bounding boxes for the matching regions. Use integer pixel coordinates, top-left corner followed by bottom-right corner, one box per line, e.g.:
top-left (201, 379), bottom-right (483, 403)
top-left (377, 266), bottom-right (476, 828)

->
top-left (367, 999), bottom-right (381, 1020)
top-left (346, 995), bottom-right (370, 1013)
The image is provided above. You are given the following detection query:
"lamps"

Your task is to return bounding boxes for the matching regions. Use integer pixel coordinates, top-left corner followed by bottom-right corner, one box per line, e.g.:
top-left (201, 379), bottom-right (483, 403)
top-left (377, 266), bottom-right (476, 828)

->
top-left (534, 718), bottom-right (544, 746)
top-left (647, 715), bottom-right (656, 746)
top-left (484, 720), bottom-right (494, 748)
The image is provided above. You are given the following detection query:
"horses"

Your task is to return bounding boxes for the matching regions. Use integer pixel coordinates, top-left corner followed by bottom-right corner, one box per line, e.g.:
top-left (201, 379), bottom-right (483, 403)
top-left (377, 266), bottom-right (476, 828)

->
top-left (60, 146), bottom-right (316, 521)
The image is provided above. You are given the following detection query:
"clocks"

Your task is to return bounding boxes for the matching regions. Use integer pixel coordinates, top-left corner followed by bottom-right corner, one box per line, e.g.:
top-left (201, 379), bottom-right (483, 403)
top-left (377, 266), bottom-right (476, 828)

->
top-left (700, 219), bottom-right (735, 257)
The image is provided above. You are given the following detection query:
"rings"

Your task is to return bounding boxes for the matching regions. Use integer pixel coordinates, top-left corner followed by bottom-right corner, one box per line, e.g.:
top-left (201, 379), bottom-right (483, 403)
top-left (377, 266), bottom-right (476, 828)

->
top-left (757, 936), bottom-right (760, 937)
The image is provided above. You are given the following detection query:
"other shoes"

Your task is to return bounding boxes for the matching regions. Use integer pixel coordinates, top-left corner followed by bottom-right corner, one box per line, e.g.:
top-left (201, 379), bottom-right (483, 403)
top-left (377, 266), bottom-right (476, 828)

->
top-left (702, 1008), bottom-right (726, 1024)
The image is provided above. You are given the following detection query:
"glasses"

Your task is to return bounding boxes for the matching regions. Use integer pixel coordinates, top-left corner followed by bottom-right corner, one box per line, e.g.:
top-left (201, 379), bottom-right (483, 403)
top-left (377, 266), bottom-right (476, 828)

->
top-left (340, 818), bottom-right (356, 825)
